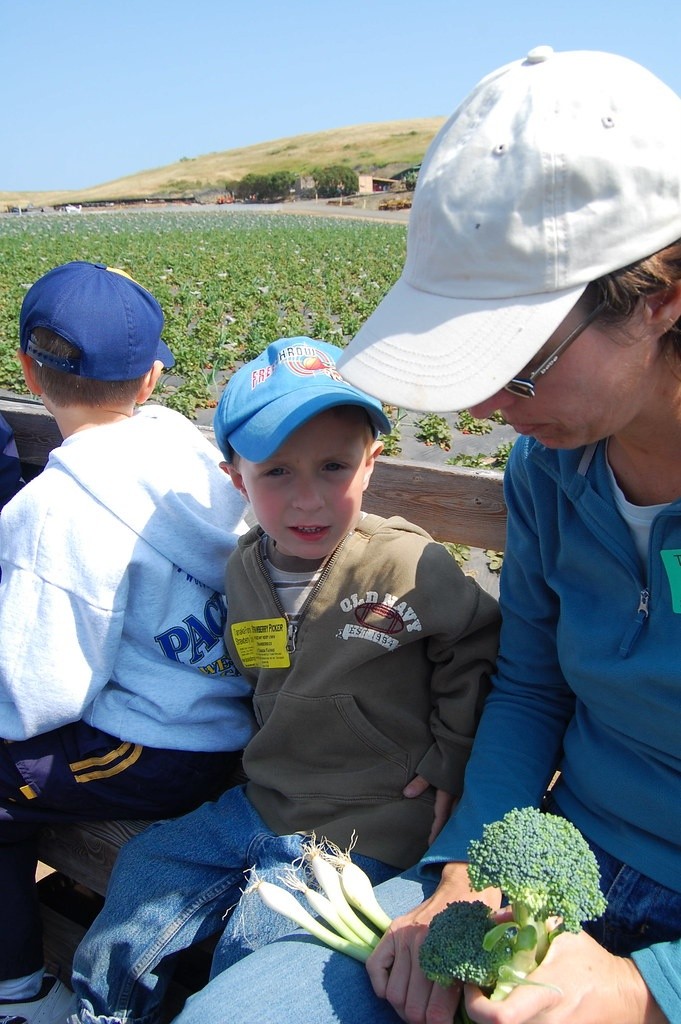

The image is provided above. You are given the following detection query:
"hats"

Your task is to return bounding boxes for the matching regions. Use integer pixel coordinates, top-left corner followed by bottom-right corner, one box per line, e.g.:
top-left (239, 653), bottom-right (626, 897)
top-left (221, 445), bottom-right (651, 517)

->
top-left (336, 45), bottom-right (681, 412)
top-left (213, 340), bottom-right (392, 465)
top-left (20, 259), bottom-right (174, 382)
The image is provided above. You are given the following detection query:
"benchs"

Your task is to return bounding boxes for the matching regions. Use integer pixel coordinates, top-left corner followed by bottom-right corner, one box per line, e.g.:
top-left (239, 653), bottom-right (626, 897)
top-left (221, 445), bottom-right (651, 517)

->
top-left (0, 390), bottom-right (563, 900)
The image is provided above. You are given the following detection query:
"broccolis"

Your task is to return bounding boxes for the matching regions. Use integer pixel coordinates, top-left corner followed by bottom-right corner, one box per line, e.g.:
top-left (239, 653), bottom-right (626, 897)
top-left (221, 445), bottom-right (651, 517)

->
top-left (416, 806), bottom-right (608, 1003)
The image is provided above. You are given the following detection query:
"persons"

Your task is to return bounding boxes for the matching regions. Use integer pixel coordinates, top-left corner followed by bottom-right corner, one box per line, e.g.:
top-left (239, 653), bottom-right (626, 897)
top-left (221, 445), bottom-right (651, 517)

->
top-left (60, 337), bottom-right (501, 1024)
top-left (175, 45), bottom-right (681, 1024)
top-left (0, 261), bottom-right (258, 1024)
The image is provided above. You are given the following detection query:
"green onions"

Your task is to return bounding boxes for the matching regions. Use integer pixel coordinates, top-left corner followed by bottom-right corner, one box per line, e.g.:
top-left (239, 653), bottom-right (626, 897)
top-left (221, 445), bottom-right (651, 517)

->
top-left (221, 830), bottom-right (394, 964)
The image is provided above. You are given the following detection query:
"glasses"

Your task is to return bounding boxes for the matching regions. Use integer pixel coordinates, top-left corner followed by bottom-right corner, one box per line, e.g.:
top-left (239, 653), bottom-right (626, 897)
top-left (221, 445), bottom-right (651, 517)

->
top-left (503, 300), bottom-right (622, 398)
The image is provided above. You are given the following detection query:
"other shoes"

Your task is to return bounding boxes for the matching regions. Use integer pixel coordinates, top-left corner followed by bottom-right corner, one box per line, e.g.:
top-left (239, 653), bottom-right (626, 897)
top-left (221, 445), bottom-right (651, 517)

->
top-left (0, 973), bottom-right (77, 1024)
